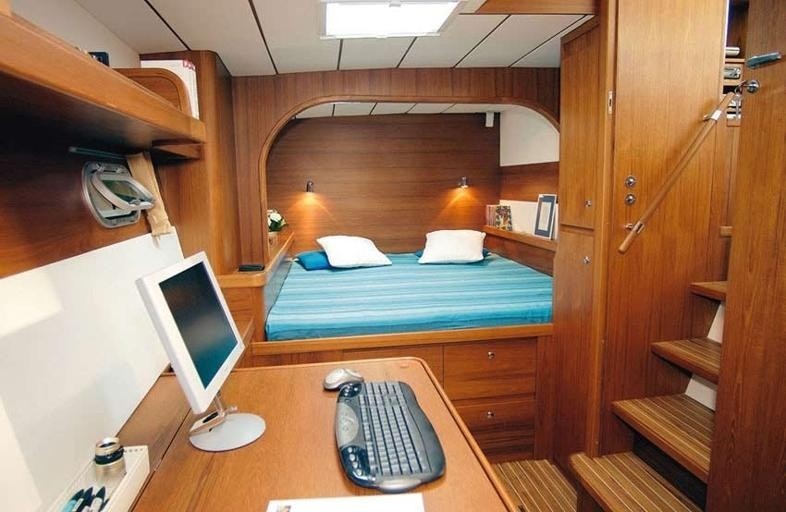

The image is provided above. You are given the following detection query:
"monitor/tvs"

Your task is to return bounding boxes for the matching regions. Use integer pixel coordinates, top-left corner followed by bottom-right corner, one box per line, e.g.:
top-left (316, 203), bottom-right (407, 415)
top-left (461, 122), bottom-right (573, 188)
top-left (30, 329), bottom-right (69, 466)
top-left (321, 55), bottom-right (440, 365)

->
top-left (134, 250), bottom-right (267, 453)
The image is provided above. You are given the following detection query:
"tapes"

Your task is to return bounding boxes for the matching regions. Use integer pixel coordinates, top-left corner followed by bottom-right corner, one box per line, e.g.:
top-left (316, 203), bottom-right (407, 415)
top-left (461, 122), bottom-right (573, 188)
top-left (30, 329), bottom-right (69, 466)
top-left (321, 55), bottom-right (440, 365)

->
top-left (92, 438), bottom-right (124, 471)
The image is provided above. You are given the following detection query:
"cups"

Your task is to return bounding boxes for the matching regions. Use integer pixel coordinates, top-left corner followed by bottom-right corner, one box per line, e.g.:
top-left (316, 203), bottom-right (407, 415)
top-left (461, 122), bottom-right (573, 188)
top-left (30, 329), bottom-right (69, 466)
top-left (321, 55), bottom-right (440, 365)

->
top-left (94, 437), bottom-right (130, 493)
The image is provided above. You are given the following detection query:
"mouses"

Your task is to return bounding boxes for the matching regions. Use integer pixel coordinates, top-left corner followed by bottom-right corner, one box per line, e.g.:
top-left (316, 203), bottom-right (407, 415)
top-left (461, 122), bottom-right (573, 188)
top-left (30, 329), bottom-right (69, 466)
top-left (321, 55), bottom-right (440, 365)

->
top-left (324, 367), bottom-right (362, 390)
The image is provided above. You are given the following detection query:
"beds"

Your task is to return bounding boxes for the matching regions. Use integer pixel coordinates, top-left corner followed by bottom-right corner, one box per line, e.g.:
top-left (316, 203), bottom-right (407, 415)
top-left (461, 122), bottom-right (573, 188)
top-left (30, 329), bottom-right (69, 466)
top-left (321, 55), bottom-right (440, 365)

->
top-left (264, 246), bottom-right (557, 463)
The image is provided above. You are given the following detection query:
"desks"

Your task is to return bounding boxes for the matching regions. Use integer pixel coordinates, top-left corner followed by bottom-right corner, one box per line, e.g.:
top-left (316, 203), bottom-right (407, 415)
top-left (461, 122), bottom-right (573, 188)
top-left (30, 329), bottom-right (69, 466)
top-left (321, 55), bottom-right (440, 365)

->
top-left (94, 355), bottom-right (518, 512)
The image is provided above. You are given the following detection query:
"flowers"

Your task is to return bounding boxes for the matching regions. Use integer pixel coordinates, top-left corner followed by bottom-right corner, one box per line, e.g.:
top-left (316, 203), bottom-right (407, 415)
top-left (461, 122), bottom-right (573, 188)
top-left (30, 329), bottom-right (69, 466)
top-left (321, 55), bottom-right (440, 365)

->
top-left (267, 207), bottom-right (289, 230)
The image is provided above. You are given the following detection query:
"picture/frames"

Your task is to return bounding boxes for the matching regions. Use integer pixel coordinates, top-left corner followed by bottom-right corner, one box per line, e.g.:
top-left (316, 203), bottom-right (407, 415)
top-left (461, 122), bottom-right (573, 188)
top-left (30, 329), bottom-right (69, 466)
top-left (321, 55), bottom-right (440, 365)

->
top-left (532, 193), bottom-right (558, 240)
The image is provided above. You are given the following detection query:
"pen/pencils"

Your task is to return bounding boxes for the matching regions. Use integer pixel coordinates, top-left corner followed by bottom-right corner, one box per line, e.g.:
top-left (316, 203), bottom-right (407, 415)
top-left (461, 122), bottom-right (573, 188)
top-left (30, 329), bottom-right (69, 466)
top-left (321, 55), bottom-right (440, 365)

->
top-left (62, 487), bottom-right (109, 512)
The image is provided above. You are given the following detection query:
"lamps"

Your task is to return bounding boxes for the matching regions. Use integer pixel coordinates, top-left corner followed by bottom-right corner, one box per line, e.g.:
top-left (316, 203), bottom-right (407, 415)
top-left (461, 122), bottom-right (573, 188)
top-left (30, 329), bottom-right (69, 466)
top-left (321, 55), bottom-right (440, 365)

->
top-left (455, 176), bottom-right (468, 189)
top-left (304, 175), bottom-right (314, 192)
top-left (320, 0), bottom-right (464, 38)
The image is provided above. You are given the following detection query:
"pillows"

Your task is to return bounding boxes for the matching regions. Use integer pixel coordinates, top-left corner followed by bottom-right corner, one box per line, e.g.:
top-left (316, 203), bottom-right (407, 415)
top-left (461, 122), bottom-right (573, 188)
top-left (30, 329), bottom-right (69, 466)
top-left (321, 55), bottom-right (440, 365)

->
top-left (418, 229), bottom-right (486, 263)
top-left (293, 249), bottom-right (330, 270)
top-left (414, 246), bottom-right (490, 256)
top-left (315, 233), bottom-right (393, 267)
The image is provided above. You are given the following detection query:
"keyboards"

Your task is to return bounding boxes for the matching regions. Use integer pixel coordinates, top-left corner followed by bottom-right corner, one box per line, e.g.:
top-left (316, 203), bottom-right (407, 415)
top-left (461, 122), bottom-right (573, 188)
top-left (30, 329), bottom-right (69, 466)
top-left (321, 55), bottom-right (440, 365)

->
top-left (335, 381), bottom-right (446, 494)
